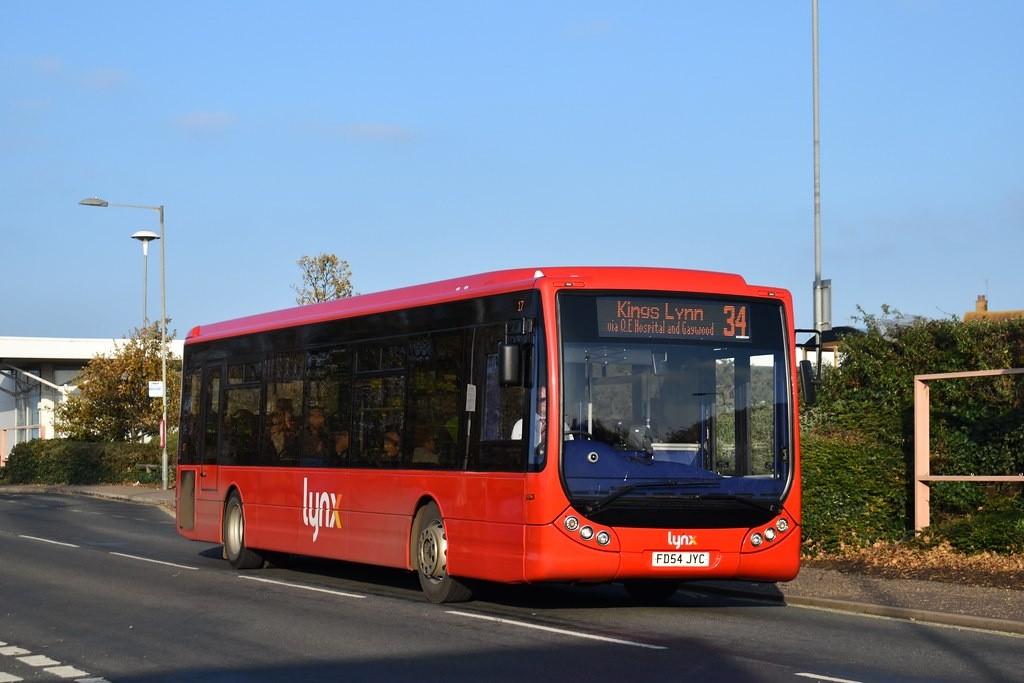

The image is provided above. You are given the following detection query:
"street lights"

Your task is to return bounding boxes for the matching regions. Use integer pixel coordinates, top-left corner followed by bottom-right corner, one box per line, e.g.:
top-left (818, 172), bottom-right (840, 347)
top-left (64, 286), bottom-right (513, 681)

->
top-left (79, 196), bottom-right (169, 491)
top-left (132, 230), bottom-right (161, 355)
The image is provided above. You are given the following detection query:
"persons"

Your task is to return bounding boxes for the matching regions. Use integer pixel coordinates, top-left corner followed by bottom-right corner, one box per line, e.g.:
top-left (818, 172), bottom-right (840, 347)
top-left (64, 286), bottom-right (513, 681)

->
top-left (183, 389), bottom-right (450, 465)
top-left (628, 399), bottom-right (674, 454)
top-left (510, 386), bottom-right (573, 453)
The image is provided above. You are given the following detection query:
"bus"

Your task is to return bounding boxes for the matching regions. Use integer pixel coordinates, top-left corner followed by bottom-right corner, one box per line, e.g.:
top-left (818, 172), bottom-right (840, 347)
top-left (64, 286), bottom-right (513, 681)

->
top-left (175, 265), bottom-right (823, 606)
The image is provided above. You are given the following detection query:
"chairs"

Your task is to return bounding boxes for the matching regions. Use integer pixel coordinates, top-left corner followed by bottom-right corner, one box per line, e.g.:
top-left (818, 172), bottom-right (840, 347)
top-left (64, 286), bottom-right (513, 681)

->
top-left (232, 409), bottom-right (258, 440)
top-left (192, 409), bottom-right (218, 434)
top-left (327, 411), bottom-right (345, 431)
